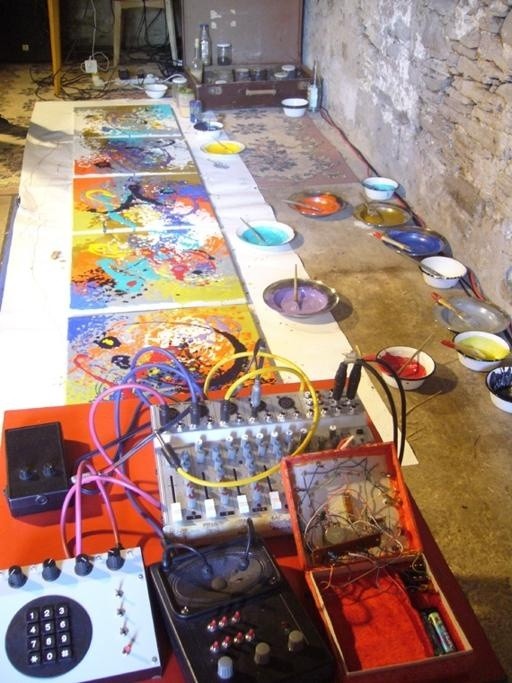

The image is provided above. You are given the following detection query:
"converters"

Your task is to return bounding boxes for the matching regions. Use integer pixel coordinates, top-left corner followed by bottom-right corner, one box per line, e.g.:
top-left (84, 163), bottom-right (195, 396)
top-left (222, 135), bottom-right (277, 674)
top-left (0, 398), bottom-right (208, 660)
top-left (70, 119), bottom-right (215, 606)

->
top-left (119, 68), bottom-right (128, 79)
top-left (85, 60), bottom-right (97, 73)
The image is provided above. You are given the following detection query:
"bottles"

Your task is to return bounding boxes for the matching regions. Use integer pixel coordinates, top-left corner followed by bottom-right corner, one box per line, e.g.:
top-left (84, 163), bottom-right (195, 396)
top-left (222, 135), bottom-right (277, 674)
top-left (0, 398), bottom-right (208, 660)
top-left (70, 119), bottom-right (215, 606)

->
top-left (177, 87), bottom-right (195, 117)
top-left (203, 64), bottom-right (295, 83)
top-left (172, 78), bottom-right (188, 105)
top-left (216, 42), bottom-right (232, 66)
top-left (188, 36), bottom-right (203, 83)
top-left (307, 55), bottom-right (324, 112)
top-left (197, 21), bottom-right (212, 66)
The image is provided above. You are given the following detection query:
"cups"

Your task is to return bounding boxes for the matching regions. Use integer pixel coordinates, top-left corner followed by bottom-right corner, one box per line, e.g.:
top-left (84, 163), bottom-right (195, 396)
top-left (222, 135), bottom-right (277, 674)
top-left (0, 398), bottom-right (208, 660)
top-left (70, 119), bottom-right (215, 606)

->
top-left (189, 100), bottom-right (202, 123)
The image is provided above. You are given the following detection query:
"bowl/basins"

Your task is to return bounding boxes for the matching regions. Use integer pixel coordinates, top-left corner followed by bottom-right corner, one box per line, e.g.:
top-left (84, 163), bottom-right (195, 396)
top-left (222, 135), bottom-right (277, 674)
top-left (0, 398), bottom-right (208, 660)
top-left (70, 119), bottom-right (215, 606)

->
top-left (451, 329), bottom-right (511, 372)
top-left (375, 345), bottom-right (436, 390)
top-left (144, 82), bottom-right (169, 98)
top-left (281, 97), bottom-right (308, 118)
top-left (361, 176), bottom-right (400, 200)
top-left (419, 256), bottom-right (469, 290)
top-left (484, 364), bottom-right (512, 413)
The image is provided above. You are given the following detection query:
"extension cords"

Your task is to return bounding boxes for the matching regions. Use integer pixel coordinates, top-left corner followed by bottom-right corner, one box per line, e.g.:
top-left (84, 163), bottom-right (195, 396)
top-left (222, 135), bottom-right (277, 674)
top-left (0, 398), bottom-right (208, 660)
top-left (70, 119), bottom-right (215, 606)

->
top-left (115, 76), bottom-right (158, 84)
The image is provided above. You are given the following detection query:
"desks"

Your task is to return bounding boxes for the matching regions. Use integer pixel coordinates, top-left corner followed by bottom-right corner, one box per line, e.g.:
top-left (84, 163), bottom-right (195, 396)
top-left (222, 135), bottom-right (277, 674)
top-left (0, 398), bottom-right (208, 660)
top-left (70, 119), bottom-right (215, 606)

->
top-left (0, 379), bottom-right (509, 683)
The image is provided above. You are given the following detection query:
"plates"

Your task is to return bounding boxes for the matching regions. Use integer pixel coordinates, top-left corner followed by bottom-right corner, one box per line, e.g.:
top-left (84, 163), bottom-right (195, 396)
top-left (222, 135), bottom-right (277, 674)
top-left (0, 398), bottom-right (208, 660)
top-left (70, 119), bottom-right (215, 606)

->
top-left (236, 221), bottom-right (296, 247)
top-left (199, 139), bottom-right (246, 155)
top-left (262, 278), bottom-right (340, 316)
top-left (285, 188), bottom-right (346, 218)
top-left (379, 226), bottom-right (448, 256)
top-left (351, 200), bottom-right (413, 227)
top-left (192, 122), bottom-right (225, 132)
top-left (431, 295), bottom-right (510, 334)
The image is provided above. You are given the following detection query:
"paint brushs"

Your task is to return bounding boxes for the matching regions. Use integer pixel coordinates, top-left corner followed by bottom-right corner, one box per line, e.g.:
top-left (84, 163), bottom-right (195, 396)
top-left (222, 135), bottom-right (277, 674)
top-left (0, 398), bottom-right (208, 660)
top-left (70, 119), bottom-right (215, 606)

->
top-left (239, 217), bottom-right (266, 244)
top-left (396, 333), bottom-right (434, 375)
top-left (294, 264), bottom-right (299, 310)
top-left (215, 138), bottom-right (232, 154)
top-left (283, 199), bottom-right (323, 211)
top-left (383, 240), bottom-right (442, 276)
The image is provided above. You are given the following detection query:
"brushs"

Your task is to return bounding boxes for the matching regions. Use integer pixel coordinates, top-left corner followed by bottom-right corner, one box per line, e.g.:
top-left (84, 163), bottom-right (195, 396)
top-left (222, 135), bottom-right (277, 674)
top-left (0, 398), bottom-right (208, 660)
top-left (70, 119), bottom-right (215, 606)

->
top-left (372, 230), bottom-right (419, 253)
top-left (358, 193), bottom-right (383, 225)
top-left (432, 292), bottom-right (481, 327)
top-left (440, 340), bottom-right (495, 361)
